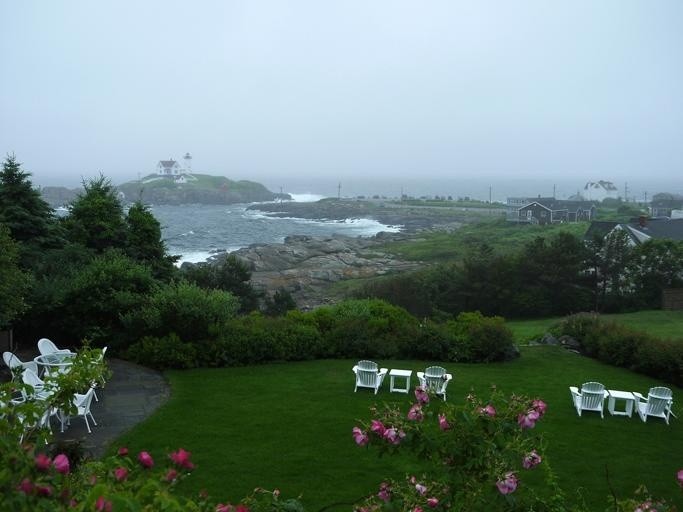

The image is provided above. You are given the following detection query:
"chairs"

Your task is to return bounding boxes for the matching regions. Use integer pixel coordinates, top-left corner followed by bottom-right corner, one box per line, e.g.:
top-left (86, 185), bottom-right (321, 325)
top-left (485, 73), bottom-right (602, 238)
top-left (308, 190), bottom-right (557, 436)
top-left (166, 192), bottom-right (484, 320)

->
top-left (352, 360), bottom-right (453, 402)
top-left (0, 338), bottom-right (109, 446)
top-left (570, 382), bottom-right (673, 426)
